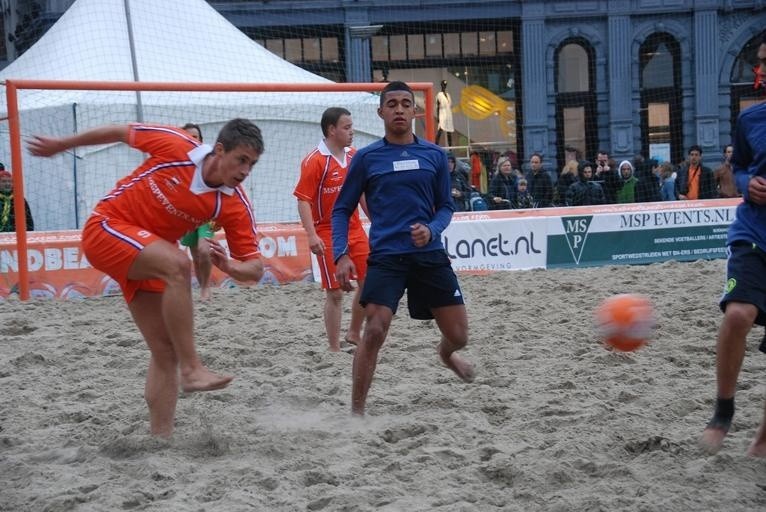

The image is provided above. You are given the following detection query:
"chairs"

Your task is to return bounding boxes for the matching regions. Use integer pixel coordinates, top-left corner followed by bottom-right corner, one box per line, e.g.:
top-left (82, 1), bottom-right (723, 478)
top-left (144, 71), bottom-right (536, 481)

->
top-left (468, 191), bottom-right (512, 210)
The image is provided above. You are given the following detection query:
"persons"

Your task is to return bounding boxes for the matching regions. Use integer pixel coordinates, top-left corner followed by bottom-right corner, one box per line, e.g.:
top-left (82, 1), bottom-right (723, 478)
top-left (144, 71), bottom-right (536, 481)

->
top-left (448, 144), bottom-right (743, 211)
top-left (23, 119), bottom-right (264, 439)
top-left (696, 37), bottom-right (766, 458)
top-left (0, 170), bottom-right (34, 232)
top-left (293, 107), bottom-right (372, 352)
top-left (435, 80), bottom-right (454, 146)
top-left (179, 124), bottom-right (221, 299)
top-left (331, 81), bottom-right (474, 418)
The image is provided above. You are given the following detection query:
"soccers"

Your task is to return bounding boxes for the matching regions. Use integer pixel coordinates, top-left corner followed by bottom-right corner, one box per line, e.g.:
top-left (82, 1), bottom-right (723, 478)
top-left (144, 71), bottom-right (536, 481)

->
top-left (593, 292), bottom-right (656, 353)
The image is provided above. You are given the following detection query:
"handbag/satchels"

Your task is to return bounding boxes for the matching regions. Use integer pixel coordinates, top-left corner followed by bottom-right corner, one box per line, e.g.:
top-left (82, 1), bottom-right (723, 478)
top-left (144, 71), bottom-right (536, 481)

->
top-left (464, 191), bottom-right (489, 211)
